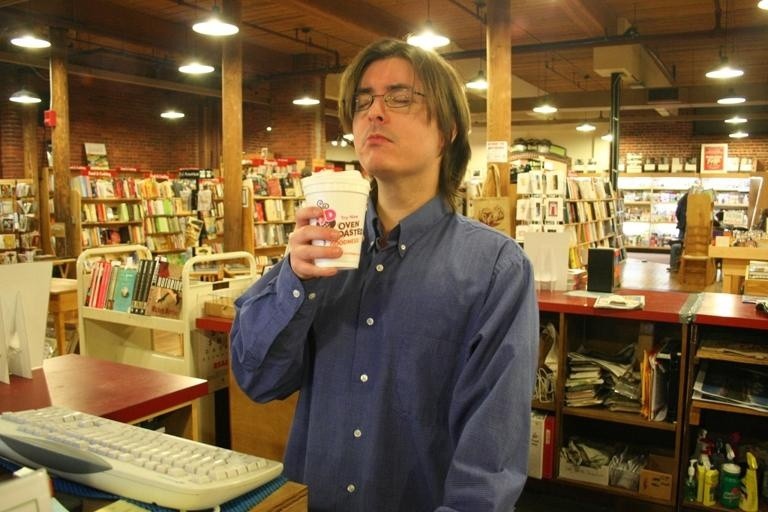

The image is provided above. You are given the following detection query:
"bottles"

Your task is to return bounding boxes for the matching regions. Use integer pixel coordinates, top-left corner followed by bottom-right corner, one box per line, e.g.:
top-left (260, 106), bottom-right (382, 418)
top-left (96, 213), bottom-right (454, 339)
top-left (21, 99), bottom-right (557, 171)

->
top-left (697, 460), bottom-right (741, 509)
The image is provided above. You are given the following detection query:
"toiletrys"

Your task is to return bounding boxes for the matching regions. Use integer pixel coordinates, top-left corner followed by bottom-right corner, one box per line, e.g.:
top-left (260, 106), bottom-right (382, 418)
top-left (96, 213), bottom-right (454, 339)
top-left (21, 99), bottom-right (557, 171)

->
top-left (683, 458), bottom-right (699, 504)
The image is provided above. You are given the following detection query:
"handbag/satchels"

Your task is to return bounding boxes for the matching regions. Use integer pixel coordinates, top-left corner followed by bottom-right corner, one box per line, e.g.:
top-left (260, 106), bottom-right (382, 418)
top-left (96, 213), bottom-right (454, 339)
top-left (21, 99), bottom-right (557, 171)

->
top-left (472, 164), bottom-right (513, 238)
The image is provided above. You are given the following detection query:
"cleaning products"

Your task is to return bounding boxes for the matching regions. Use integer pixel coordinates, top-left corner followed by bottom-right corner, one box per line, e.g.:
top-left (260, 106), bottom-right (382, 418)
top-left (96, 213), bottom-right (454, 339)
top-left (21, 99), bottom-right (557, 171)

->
top-left (739, 452), bottom-right (759, 512)
top-left (695, 430), bottom-right (719, 508)
top-left (724, 441), bottom-right (736, 463)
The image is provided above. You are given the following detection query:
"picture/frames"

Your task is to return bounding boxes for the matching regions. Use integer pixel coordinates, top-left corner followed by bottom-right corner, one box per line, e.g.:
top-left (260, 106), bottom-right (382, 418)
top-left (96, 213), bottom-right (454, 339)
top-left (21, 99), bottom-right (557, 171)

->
top-left (700, 143), bottom-right (728, 175)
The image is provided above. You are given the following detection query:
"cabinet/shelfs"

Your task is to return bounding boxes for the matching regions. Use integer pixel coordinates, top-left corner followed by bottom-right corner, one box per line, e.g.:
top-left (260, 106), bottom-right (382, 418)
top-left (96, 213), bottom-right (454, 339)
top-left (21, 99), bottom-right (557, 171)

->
top-left (511, 172), bottom-right (768, 296)
top-left (75, 244), bottom-right (258, 446)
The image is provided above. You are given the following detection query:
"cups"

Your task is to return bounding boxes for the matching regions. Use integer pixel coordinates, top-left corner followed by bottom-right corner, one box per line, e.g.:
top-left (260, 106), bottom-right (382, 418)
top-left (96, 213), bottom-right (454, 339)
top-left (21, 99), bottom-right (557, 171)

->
top-left (299, 169), bottom-right (371, 270)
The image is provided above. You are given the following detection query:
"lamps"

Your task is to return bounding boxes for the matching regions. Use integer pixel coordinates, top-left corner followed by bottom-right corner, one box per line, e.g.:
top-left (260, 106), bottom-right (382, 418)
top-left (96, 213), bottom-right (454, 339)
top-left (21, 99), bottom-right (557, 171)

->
top-left (9, 0), bottom-right (52, 49)
top-left (405, 0), bottom-right (451, 52)
top-left (532, 47), bottom-right (558, 115)
top-left (575, 75), bottom-right (597, 133)
top-left (703, 0), bottom-right (750, 139)
top-left (291, 25), bottom-right (321, 106)
top-left (600, 118), bottom-right (614, 142)
top-left (464, 0), bottom-right (490, 91)
top-left (159, 0), bottom-right (240, 120)
top-left (8, 83), bottom-right (43, 104)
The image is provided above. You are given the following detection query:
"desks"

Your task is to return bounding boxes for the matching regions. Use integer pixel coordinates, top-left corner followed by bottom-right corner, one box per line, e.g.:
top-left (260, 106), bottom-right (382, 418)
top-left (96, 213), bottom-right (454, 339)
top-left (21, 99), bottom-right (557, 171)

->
top-left (195, 314), bottom-right (301, 465)
top-left (0, 353), bottom-right (210, 443)
top-left (45, 278), bottom-right (78, 355)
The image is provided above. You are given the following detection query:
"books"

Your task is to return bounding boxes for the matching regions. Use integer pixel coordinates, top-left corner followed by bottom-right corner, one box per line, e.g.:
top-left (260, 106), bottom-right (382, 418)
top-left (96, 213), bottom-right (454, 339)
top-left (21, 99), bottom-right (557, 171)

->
top-left (239, 156), bottom-right (354, 275)
top-left (0, 156), bottom-right (43, 263)
top-left (747, 260), bottom-right (768, 282)
top-left (558, 319), bottom-right (665, 421)
top-left (69, 164), bottom-right (224, 318)
top-left (49, 174), bottom-right (55, 217)
top-left (511, 138), bottom-right (629, 293)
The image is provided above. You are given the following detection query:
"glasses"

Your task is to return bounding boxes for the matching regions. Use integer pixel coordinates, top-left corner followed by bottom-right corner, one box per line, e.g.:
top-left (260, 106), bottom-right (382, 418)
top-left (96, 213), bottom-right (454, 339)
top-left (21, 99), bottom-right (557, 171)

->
top-left (351, 85), bottom-right (428, 111)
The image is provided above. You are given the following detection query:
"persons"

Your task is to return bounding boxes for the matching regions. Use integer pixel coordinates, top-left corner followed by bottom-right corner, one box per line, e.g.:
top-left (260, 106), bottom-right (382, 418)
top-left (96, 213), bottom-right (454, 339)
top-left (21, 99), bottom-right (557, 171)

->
top-left (675, 189), bottom-right (687, 240)
top-left (229, 35), bottom-right (541, 512)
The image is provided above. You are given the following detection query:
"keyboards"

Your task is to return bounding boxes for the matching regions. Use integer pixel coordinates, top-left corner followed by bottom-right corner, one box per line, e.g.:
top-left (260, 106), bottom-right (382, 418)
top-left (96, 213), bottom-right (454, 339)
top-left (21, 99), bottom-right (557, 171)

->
top-left (0, 406), bottom-right (284, 510)
